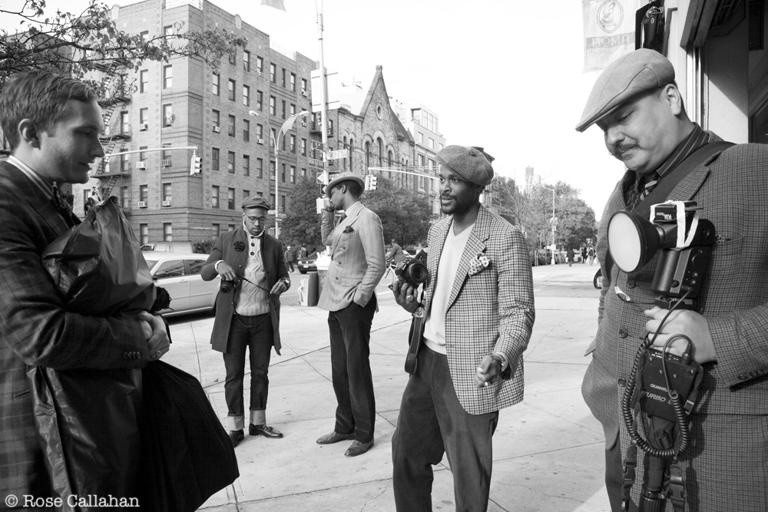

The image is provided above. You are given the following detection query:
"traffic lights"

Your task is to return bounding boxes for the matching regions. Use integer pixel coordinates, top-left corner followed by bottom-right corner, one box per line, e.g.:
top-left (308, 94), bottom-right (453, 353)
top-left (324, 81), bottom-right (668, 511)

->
top-left (186, 149), bottom-right (206, 180)
top-left (365, 174), bottom-right (377, 192)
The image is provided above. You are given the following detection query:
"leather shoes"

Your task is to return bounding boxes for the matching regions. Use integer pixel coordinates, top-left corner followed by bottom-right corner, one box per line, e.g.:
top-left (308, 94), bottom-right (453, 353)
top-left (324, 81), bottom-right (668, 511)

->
top-left (229, 430), bottom-right (245, 447)
top-left (249, 425), bottom-right (281, 438)
top-left (317, 431), bottom-right (354, 444)
top-left (345, 439), bottom-right (373, 456)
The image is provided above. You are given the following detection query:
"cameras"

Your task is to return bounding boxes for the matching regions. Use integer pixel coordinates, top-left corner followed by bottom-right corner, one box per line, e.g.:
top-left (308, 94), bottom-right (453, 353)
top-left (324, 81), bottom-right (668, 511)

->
top-left (220, 278), bottom-right (235, 293)
top-left (394, 249), bottom-right (427, 289)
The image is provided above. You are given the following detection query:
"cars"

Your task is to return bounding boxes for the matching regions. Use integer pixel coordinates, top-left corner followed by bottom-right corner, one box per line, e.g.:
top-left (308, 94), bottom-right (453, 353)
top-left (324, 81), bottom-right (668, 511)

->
top-left (134, 251), bottom-right (233, 325)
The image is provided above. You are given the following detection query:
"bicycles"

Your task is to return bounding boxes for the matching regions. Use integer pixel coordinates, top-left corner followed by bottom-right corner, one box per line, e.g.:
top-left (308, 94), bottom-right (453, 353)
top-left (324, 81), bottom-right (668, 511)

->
top-left (379, 256), bottom-right (415, 287)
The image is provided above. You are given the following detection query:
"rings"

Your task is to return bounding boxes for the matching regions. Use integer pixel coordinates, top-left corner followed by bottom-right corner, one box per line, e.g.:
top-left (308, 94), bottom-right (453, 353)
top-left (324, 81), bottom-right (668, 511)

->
top-left (155, 350), bottom-right (161, 357)
top-left (485, 382), bottom-right (489, 388)
top-left (406, 295), bottom-right (414, 303)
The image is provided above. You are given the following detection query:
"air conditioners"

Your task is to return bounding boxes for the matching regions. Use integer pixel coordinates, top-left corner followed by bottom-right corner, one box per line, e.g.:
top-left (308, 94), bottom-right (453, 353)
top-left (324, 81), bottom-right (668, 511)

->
top-left (302, 123), bottom-right (307, 127)
top-left (161, 160), bottom-right (170, 166)
top-left (258, 139), bottom-right (264, 144)
top-left (139, 124), bottom-right (147, 130)
top-left (162, 201), bottom-right (170, 206)
top-left (302, 91), bottom-right (307, 96)
top-left (213, 126), bottom-right (220, 132)
top-left (136, 162), bottom-right (145, 168)
top-left (138, 202), bottom-right (145, 208)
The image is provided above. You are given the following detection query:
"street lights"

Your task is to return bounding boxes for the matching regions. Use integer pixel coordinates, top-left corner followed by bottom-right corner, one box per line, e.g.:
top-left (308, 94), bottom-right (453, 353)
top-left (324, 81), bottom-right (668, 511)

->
top-left (247, 108), bottom-right (279, 239)
top-left (528, 178), bottom-right (559, 267)
top-left (255, 0), bottom-right (338, 282)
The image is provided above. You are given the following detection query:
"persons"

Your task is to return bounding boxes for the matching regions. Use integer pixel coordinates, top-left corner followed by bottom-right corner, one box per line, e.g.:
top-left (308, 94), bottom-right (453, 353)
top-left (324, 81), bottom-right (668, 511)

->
top-left (285, 246), bottom-right (294, 272)
top-left (0, 72), bottom-right (170, 511)
top-left (201, 196), bottom-right (291, 447)
top-left (387, 239), bottom-right (404, 264)
top-left (574, 48), bottom-right (767, 511)
top-left (316, 173), bottom-right (385, 457)
top-left (392, 145), bottom-right (536, 511)
top-left (566, 248), bottom-right (599, 264)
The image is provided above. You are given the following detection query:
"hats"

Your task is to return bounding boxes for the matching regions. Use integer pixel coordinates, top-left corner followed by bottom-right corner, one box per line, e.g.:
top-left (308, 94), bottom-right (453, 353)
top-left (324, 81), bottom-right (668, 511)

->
top-left (242, 195), bottom-right (271, 210)
top-left (326, 173), bottom-right (365, 197)
top-left (576, 48), bottom-right (675, 131)
top-left (437, 145), bottom-right (494, 185)
